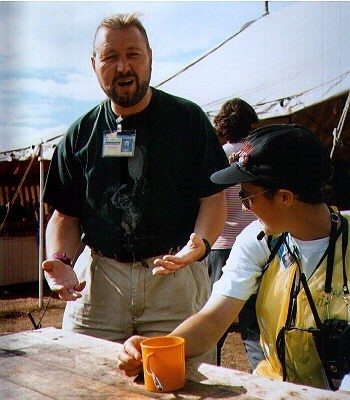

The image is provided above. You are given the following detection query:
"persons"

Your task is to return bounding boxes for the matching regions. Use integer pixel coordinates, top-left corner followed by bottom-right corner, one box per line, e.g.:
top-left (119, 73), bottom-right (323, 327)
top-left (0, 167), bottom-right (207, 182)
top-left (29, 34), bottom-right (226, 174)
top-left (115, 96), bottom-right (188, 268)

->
top-left (206, 98), bottom-right (266, 372)
top-left (43, 13), bottom-right (239, 369)
top-left (114, 125), bottom-right (350, 392)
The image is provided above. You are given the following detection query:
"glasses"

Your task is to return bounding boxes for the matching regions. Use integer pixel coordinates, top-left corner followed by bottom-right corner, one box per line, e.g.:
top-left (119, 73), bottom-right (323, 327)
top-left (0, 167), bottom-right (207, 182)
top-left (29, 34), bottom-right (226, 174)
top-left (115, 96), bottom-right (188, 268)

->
top-left (238, 189), bottom-right (271, 209)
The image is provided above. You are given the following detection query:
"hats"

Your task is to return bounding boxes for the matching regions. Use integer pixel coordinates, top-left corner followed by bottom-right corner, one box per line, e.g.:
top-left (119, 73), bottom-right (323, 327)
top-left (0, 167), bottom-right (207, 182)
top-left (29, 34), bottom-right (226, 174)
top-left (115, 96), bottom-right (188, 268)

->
top-left (210, 123), bottom-right (334, 198)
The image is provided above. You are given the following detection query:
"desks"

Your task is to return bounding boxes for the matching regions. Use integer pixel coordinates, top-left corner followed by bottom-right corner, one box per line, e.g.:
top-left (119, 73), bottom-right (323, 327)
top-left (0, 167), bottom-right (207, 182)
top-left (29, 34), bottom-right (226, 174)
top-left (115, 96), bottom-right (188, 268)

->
top-left (0, 327), bottom-right (350, 400)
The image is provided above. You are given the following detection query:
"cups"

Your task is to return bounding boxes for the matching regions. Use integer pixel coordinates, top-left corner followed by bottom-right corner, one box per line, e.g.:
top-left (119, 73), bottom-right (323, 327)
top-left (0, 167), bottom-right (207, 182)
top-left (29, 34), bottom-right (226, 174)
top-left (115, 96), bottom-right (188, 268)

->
top-left (140, 336), bottom-right (186, 393)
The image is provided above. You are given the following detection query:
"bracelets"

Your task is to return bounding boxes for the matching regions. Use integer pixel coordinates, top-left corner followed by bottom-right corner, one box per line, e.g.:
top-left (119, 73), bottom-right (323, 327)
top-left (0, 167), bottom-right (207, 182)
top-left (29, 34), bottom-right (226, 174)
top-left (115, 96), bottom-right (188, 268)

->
top-left (196, 237), bottom-right (212, 263)
top-left (47, 253), bottom-right (74, 267)
